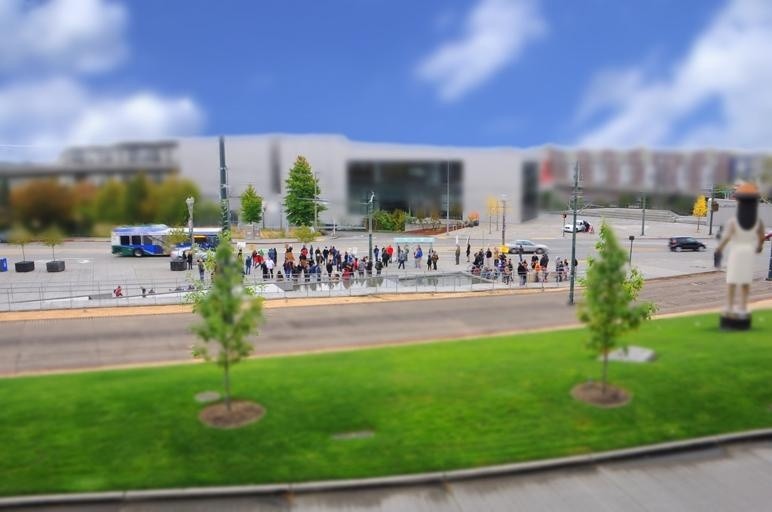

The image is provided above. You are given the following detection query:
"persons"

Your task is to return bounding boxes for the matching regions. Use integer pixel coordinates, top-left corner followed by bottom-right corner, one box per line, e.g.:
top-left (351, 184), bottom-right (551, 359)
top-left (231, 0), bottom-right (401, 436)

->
top-left (580, 220), bottom-right (593, 233)
top-left (197, 257), bottom-right (207, 283)
top-left (454, 243), bottom-right (461, 266)
top-left (465, 242), bottom-right (578, 286)
top-left (713, 181), bottom-right (766, 316)
top-left (187, 251), bottom-right (193, 270)
top-left (113, 285), bottom-right (123, 297)
top-left (181, 251), bottom-right (187, 260)
top-left (235, 242), bottom-right (440, 281)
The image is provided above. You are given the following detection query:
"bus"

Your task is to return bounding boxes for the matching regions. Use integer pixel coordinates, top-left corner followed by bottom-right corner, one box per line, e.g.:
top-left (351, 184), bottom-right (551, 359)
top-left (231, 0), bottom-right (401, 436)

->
top-left (109, 223), bottom-right (222, 258)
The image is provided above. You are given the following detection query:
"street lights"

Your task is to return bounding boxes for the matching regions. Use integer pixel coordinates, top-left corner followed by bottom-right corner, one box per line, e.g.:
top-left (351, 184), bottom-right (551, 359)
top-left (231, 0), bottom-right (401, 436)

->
top-left (184, 192), bottom-right (196, 253)
top-left (501, 192), bottom-right (508, 247)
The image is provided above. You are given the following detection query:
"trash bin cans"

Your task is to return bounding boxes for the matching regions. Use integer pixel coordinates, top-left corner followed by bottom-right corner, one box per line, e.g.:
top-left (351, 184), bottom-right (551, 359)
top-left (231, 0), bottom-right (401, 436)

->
top-left (0, 258), bottom-right (8, 272)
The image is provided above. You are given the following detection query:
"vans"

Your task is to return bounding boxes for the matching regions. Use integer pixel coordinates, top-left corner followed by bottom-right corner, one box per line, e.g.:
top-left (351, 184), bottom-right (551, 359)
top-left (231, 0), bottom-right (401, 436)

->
top-left (666, 235), bottom-right (707, 253)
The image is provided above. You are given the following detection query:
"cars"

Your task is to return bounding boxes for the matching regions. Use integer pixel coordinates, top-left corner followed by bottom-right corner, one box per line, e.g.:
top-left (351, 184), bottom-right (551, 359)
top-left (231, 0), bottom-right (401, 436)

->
top-left (505, 239), bottom-right (550, 255)
top-left (562, 219), bottom-right (590, 234)
top-left (171, 246), bottom-right (218, 265)
